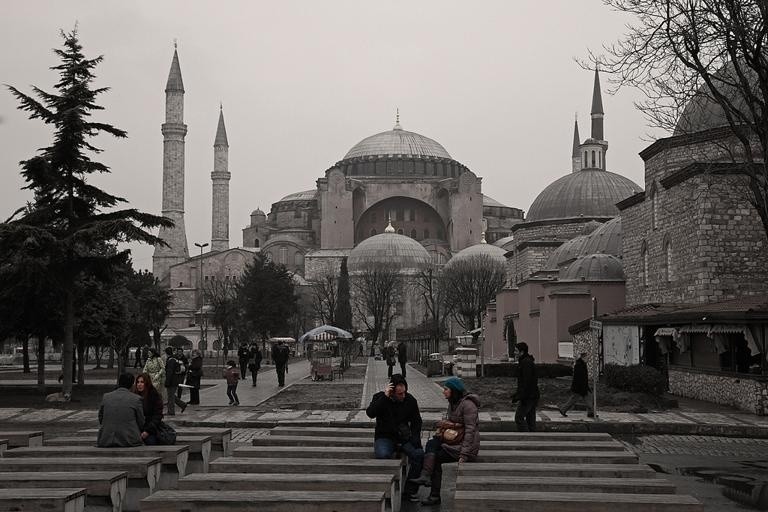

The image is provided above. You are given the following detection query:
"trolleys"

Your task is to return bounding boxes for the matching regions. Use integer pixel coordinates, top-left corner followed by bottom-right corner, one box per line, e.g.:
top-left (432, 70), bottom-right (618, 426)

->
top-left (309, 349), bottom-right (333, 382)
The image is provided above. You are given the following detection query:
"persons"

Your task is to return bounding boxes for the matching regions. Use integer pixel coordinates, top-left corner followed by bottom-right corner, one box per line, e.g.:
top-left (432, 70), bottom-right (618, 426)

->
top-left (559, 353), bottom-right (599, 418)
top-left (373, 340), bottom-right (407, 378)
top-left (510, 342), bottom-right (540, 432)
top-left (248, 343), bottom-right (262, 386)
top-left (358, 344), bottom-right (363, 357)
top-left (410, 376), bottom-right (480, 507)
top-left (366, 374), bottom-right (425, 501)
top-left (223, 360), bottom-right (240, 406)
top-left (222, 343), bottom-right (228, 360)
top-left (237, 342), bottom-right (249, 380)
top-left (98, 344), bottom-right (203, 450)
top-left (58, 344), bottom-right (65, 384)
top-left (271, 340), bottom-right (290, 387)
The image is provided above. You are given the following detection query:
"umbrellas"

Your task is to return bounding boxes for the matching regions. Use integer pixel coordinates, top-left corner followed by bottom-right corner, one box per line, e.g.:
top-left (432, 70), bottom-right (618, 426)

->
top-left (299, 323), bottom-right (352, 350)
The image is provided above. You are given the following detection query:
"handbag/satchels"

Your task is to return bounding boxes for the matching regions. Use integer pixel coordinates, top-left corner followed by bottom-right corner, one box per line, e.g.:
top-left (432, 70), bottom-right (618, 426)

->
top-left (435, 420), bottom-right (465, 445)
top-left (158, 421), bottom-right (177, 444)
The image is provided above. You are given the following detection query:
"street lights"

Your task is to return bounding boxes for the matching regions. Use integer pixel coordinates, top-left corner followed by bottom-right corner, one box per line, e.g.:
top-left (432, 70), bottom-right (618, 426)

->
top-left (194, 242), bottom-right (210, 356)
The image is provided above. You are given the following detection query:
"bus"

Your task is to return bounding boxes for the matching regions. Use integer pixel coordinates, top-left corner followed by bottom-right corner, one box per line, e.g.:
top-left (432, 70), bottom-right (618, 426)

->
top-left (0, 344), bottom-right (31, 364)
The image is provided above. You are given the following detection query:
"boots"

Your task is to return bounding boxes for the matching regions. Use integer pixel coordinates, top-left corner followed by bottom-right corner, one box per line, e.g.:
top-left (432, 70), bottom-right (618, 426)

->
top-left (409, 453), bottom-right (435, 487)
top-left (421, 472), bottom-right (442, 505)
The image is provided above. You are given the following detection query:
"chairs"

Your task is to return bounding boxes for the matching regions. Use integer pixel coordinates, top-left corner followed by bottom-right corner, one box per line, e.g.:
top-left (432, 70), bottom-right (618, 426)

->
top-left (333, 360), bottom-right (345, 380)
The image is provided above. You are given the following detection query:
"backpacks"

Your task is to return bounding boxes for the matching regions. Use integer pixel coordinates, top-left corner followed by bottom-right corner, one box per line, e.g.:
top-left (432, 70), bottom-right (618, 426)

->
top-left (168, 357), bottom-right (186, 375)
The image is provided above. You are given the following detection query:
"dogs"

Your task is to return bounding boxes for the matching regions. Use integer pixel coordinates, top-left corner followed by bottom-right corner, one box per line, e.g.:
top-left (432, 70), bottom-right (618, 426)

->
top-left (45, 390), bottom-right (72, 403)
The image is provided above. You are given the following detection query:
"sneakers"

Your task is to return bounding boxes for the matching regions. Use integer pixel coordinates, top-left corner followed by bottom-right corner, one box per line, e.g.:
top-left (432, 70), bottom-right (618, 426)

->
top-left (587, 414), bottom-right (599, 418)
top-left (163, 412), bottom-right (175, 416)
top-left (408, 493), bottom-right (420, 502)
top-left (181, 403), bottom-right (188, 413)
top-left (560, 410), bottom-right (568, 417)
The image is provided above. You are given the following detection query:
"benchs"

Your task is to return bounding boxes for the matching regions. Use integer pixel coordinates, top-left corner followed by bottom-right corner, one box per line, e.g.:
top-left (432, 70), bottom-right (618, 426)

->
top-left (0, 424), bottom-right (710, 511)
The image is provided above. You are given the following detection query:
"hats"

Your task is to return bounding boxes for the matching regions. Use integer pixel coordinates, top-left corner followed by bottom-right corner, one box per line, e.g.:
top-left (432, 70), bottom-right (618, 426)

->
top-left (443, 376), bottom-right (464, 394)
